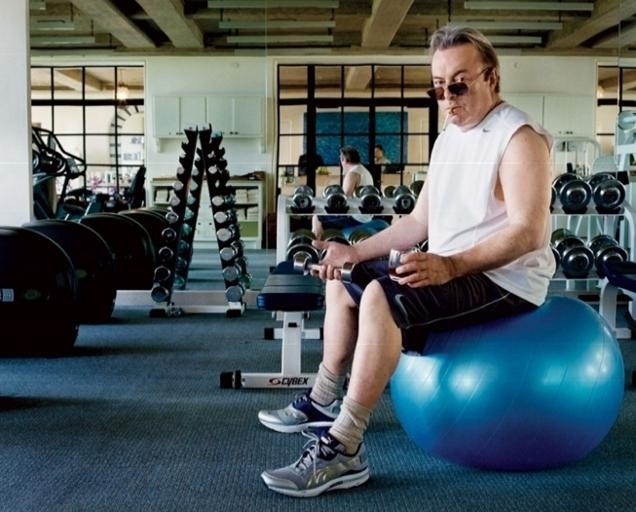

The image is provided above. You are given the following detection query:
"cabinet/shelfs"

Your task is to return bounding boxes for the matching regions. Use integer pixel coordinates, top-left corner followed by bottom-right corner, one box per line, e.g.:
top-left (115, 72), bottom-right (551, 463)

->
top-left (284, 192), bottom-right (633, 341)
top-left (151, 91), bottom-right (268, 153)
top-left (147, 178), bottom-right (266, 253)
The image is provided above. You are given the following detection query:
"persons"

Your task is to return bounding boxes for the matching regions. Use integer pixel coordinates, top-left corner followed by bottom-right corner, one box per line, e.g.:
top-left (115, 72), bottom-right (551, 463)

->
top-left (372, 145), bottom-right (392, 165)
top-left (252, 20), bottom-right (563, 499)
top-left (307, 146), bottom-right (374, 240)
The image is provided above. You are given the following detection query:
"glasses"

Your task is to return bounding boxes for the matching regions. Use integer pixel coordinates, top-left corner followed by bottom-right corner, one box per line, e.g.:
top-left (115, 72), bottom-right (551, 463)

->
top-left (426, 67), bottom-right (488, 100)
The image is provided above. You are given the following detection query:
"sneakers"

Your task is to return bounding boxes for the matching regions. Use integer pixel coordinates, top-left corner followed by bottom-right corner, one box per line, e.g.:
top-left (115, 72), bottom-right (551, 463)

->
top-left (260, 430), bottom-right (371, 498)
top-left (257, 387), bottom-right (343, 434)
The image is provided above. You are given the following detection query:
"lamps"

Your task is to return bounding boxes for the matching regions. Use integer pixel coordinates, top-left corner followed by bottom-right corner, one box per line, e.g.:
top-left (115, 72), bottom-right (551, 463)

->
top-left (113, 67), bottom-right (130, 101)
top-left (27, 0), bottom-right (118, 58)
top-left (424, 0), bottom-right (596, 49)
top-left (206, 0), bottom-right (342, 56)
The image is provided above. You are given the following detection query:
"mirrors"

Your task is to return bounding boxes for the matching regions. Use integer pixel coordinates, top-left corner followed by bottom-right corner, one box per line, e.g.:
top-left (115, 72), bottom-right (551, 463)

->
top-left (272, 63), bottom-right (447, 196)
top-left (597, 63), bottom-right (635, 152)
top-left (28, 66), bottom-right (147, 200)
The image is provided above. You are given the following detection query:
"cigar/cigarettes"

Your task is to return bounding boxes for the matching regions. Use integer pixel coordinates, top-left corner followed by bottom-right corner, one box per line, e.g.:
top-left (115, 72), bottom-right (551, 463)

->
top-left (442, 108), bottom-right (453, 132)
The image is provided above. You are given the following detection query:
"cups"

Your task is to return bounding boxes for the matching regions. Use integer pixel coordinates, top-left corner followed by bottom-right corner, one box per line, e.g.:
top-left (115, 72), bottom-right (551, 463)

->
top-left (388, 246), bottom-right (419, 282)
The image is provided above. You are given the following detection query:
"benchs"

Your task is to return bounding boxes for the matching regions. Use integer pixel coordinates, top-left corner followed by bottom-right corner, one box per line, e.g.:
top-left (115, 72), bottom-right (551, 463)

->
top-left (216, 269), bottom-right (353, 392)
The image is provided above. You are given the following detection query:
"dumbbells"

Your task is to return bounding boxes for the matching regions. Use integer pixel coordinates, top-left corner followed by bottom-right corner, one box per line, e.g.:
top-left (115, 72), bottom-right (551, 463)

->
top-left (550, 173), bottom-right (626, 214)
top-left (550, 228), bottom-right (627, 279)
top-left (287, 181), bottom-right (427, 284)
top-left (150, 122), bottom-right (252, 302)
